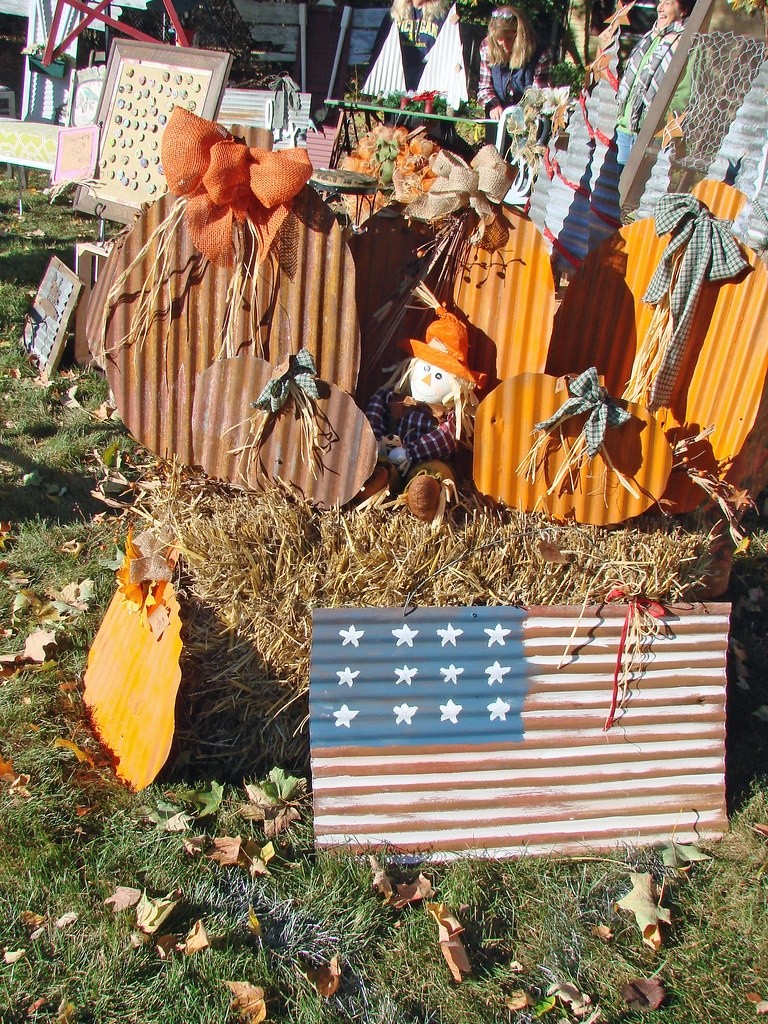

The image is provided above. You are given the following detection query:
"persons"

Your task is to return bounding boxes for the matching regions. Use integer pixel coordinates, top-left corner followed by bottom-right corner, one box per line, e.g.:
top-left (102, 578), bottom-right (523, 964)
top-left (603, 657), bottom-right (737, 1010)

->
top-left (615, 0), bottom-right (712, 179)
top-left (369, 0), bottom-right (450, 93)
top-left (476, 5), bottom-right (552, 144)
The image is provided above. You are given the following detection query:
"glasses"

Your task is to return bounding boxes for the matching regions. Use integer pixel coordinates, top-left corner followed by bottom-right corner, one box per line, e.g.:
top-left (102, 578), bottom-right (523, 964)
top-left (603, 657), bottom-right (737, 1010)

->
top-left (492, 11), bottom-right (518, 23)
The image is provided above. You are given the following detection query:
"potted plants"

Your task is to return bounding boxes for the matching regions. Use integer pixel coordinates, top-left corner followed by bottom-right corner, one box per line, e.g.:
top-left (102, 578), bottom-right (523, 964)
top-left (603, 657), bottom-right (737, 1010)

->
top-left (20, 43), bottom-right (68, 78)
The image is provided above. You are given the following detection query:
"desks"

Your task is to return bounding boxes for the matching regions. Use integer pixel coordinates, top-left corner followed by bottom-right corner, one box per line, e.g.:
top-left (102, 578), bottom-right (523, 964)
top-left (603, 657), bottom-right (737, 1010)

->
top-left (321, 93), bottom-right (498, 167)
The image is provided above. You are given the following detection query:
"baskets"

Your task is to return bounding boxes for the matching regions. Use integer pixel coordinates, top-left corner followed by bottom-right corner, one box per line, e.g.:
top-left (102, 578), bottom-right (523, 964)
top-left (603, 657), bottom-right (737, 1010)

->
top-left (402, 140), bottom-right (517, 226)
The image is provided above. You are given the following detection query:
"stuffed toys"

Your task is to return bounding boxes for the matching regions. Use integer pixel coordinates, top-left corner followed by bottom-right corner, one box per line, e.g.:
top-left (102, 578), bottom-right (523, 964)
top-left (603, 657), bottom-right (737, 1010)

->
top-left (355, 303), bottom-right (487, 523)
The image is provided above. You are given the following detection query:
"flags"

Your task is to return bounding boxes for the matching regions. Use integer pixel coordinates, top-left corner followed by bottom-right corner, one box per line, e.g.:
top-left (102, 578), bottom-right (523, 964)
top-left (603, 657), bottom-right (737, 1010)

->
top-left (310, 601), bottom-right (732, 868)
